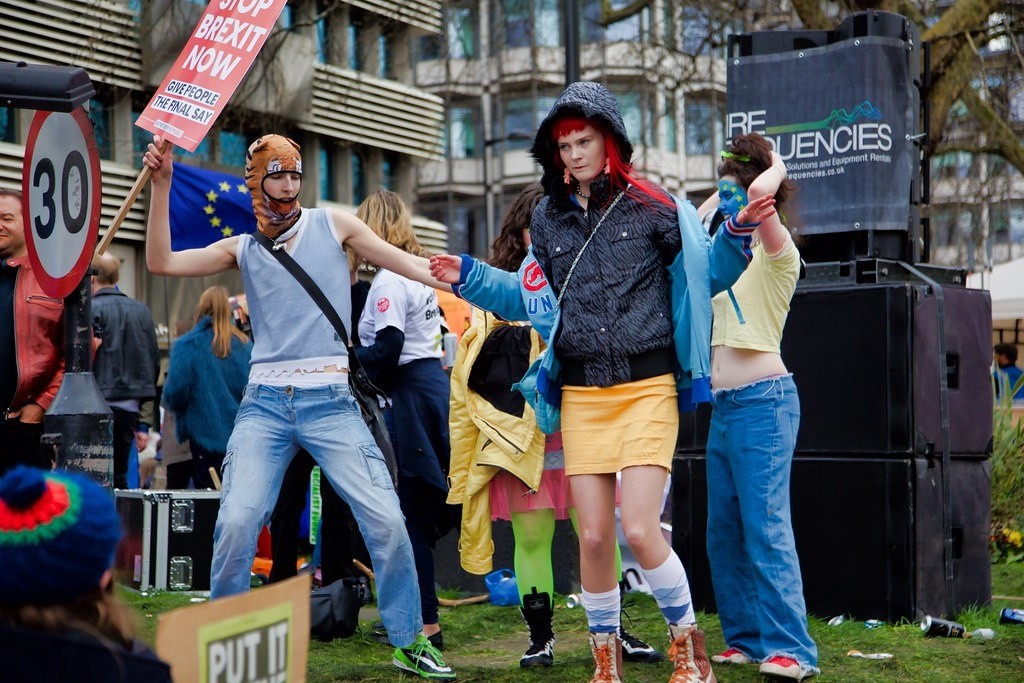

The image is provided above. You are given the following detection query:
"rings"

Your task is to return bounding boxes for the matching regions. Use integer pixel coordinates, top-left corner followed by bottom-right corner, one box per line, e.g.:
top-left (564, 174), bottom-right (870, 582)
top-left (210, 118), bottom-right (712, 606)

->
top-left (757, 209), bottom-right (762, 214)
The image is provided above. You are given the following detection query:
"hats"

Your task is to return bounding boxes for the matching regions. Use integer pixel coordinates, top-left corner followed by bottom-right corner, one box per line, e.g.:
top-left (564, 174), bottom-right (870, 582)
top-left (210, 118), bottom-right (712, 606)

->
top-left (0, 463), bottom-right (125, 603)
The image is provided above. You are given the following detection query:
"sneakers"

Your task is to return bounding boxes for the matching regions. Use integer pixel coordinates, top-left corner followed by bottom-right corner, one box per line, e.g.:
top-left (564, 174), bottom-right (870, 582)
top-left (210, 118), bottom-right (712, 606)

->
top-left (710, 647), bottom-right (751, 663)
top-left (758, 655), bottom-right (814, 678)
top-left (393, 635), bottom-right (457, 680)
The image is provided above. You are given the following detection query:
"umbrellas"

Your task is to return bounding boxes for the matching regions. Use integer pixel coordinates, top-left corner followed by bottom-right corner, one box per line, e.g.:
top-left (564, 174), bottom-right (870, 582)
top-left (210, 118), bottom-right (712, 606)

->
top-left (966, 257), bottom-right (1024, 320)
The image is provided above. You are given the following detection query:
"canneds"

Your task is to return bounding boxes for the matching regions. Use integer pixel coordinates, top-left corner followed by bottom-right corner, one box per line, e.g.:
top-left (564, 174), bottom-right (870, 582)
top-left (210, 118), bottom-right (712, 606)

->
top-left (999, 607), bottom-right (1024, 623)
top-left (920, 615), bottom-right (967, 638)
top-left (566, 593), bottom-right (582, 608)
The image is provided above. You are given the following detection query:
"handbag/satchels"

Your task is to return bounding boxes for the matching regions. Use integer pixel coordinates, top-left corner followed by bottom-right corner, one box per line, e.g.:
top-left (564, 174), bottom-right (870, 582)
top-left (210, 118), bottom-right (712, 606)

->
top-left (347, 350), bottom-right (400, 487)
top-left (511, 354), bottom-right (561, 436)
top-left (302, 576), bottom-right (362, 641)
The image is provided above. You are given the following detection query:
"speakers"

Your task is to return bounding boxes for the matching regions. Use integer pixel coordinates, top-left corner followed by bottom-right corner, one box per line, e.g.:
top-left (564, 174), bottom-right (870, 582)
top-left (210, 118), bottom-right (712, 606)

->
top-left (745, 11), bottom-right (922, 258)
top-left (663, 282), bottom-right (995, 621)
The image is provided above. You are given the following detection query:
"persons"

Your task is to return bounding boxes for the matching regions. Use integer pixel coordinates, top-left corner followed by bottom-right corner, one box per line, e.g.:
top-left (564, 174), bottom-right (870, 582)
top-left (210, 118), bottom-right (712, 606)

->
top-left (488, 182), bottom-right (664, 669)
top-left (991, 343), bottom-right (1024, 401)
top-left (161, 286), bottom-right (253, 591)
top-left (89, 253), bottom-right (161, 490)
top-left (427, 81), bottom-right (778, 683)
top-left (0, 464), bottom-right (175, 683)
top-left (697, 132), bottom-right (820, 682)
top-left (142, 134), bottom-right (454, 679)
top-left (0, 188), bottom-right (68, 479)
top-left (346, 190), bottom-right (460, 650)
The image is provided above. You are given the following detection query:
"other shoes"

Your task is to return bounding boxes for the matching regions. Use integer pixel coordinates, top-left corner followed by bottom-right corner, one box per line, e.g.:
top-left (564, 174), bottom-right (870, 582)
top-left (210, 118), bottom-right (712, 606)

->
top-left (365, 628), bottom-right (444, 650)
top-left (370, 622), bottom-right (388, 632)
top-left (356, 574), bottom-right (374, 602)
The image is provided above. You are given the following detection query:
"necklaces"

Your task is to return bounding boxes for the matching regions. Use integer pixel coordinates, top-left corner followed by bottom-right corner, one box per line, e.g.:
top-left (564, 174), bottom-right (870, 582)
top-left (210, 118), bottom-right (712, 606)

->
top-left (577, 184), bottom-right (589, 198)
top-left (750, 239), bottom-right (760, 249)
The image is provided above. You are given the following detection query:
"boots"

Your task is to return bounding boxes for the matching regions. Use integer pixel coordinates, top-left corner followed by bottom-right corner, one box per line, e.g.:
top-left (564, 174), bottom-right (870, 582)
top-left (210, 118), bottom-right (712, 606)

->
top-left (617, 569), bottom-right (666, 663)
top-left (665, 622), bottom-right (718, 683)
top-left (586, 627), bottom-right (626, 683)
top-left (517, 586), bottom-right (556, 668)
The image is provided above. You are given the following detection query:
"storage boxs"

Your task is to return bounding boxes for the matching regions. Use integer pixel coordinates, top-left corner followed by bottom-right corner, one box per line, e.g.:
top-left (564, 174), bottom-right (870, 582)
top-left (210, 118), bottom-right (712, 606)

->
top-left (116, 490), bottom-right (222, 591)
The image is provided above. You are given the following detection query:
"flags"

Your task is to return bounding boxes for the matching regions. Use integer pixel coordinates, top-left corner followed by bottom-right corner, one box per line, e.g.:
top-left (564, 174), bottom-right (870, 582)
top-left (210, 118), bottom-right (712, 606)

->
top-left (168, 160), bottom-right (257, 252)
